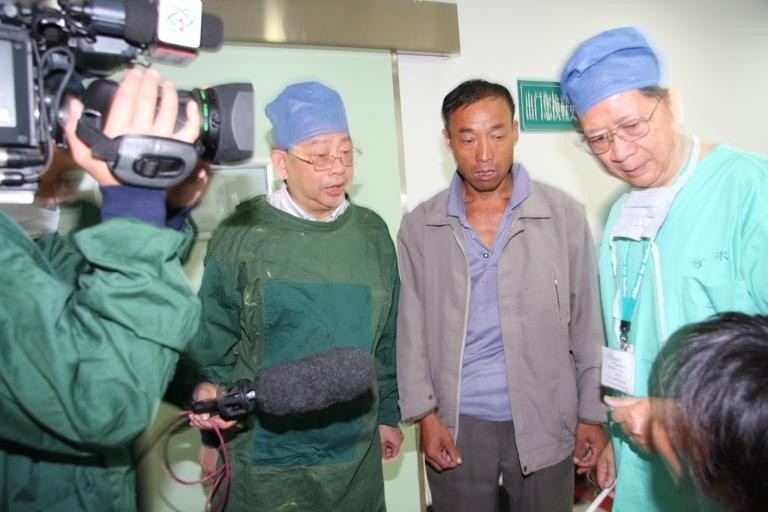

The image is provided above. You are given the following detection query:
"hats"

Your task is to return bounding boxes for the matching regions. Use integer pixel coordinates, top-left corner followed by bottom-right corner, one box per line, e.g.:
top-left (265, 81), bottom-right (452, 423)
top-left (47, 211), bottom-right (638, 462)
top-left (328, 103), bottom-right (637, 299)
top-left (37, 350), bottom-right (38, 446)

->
top-left (263, 80), bottom-right (350, 151)
top-left (559, 25), bottom-right (661, 122)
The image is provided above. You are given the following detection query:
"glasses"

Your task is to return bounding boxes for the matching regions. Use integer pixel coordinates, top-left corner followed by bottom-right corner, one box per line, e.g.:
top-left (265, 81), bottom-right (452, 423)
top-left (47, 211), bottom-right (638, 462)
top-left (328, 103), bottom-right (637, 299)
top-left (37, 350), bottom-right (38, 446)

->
top-left (286, 148), bottom-right (363, 172)
top-left (580, 96), bottom-right (664, 157)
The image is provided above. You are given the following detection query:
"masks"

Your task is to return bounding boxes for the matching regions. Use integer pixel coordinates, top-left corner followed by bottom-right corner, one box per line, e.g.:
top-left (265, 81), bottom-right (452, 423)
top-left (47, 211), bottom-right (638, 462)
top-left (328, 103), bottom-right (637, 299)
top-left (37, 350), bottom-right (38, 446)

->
top-left (609, 134), bottom-right (702, 244)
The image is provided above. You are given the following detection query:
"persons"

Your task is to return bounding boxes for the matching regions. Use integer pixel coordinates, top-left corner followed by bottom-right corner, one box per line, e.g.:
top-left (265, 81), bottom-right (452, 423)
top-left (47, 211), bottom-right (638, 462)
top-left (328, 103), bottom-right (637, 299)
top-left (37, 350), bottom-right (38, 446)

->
top-left (1, 64), bottom-right (212, 512)
top-left (188, 80), bottom-right (404, 512)
top-left (646, 311), bottom-right (768, 511)
top-left (558, 25), bottom-right (768, 512)
top-left (392, 77), bottom-right (608, 512)
